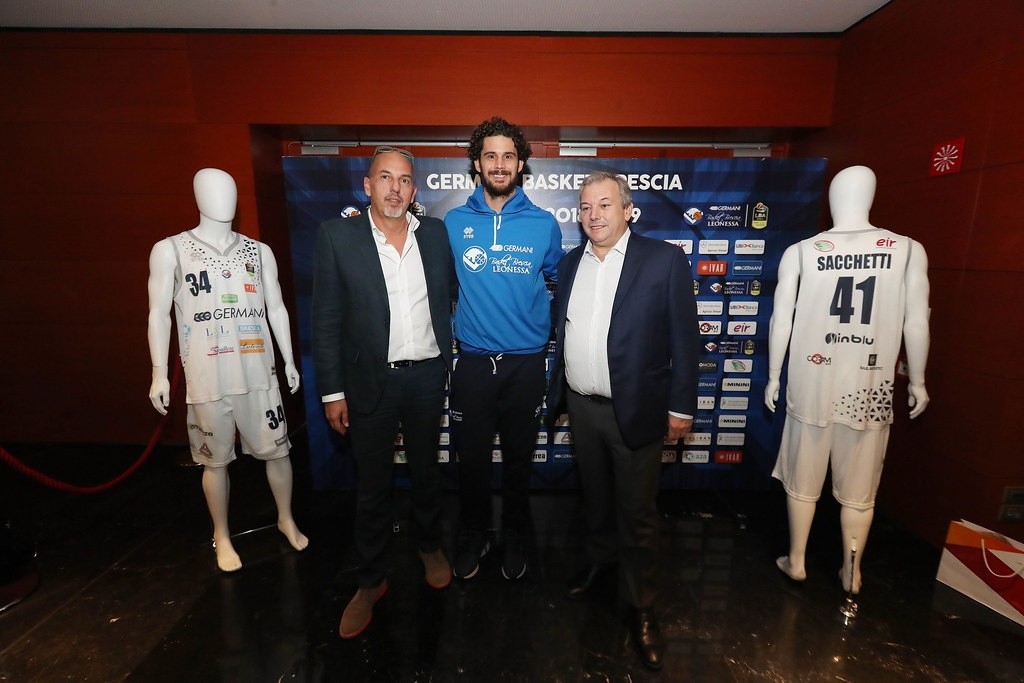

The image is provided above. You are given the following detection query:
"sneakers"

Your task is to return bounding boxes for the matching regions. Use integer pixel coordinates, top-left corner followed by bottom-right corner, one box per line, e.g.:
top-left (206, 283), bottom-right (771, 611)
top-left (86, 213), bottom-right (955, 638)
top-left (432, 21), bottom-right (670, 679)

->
top-left (501, 532), bottom-right (528, 579)
top-left (451, 534), bottom-right (491, 581)
top-left (338, 577), bottom-right (384, 637)
top-left (420, 546), bottom-right (451, 589)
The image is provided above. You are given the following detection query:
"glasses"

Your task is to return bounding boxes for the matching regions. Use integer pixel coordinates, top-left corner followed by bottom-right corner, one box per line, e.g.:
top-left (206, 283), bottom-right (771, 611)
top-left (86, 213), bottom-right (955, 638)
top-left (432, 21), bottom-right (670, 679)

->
top-left (371, 146), bottom-right (415, 167)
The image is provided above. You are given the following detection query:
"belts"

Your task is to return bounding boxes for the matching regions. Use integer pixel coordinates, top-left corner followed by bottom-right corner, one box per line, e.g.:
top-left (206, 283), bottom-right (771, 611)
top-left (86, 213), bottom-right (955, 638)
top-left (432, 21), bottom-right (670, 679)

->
top-left (387, 354), bottom-right (442, 368)
top-left (584, 393), bottom-right (613, 405)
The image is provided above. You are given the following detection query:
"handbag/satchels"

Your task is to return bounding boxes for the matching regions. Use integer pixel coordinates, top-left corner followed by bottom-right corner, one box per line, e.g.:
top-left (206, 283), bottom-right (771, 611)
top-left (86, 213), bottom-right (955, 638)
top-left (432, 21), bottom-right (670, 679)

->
top-left (934, 518), bottom-right (1024, 623)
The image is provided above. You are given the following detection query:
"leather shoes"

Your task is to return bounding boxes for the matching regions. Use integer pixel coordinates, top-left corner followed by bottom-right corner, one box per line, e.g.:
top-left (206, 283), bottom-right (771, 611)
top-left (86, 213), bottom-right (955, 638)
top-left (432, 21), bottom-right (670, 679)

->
top-left (632, 604), bottom-right (665, 670)
top-left (567, 559), bottom-right (610, 598)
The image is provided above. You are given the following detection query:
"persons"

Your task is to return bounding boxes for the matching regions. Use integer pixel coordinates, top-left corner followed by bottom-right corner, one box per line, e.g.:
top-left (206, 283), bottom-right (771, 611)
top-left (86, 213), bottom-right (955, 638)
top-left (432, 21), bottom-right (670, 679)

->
top-left (443, 117), bottom-right (568, 585)
top-left (545, 171), bottom-right (697, 671)
top-left (762, 167), bottom-right (937, 596)
top-left (306, 144), bottom-right (458, 638)
top-left (142, 167), bottom-right (310, 569)
